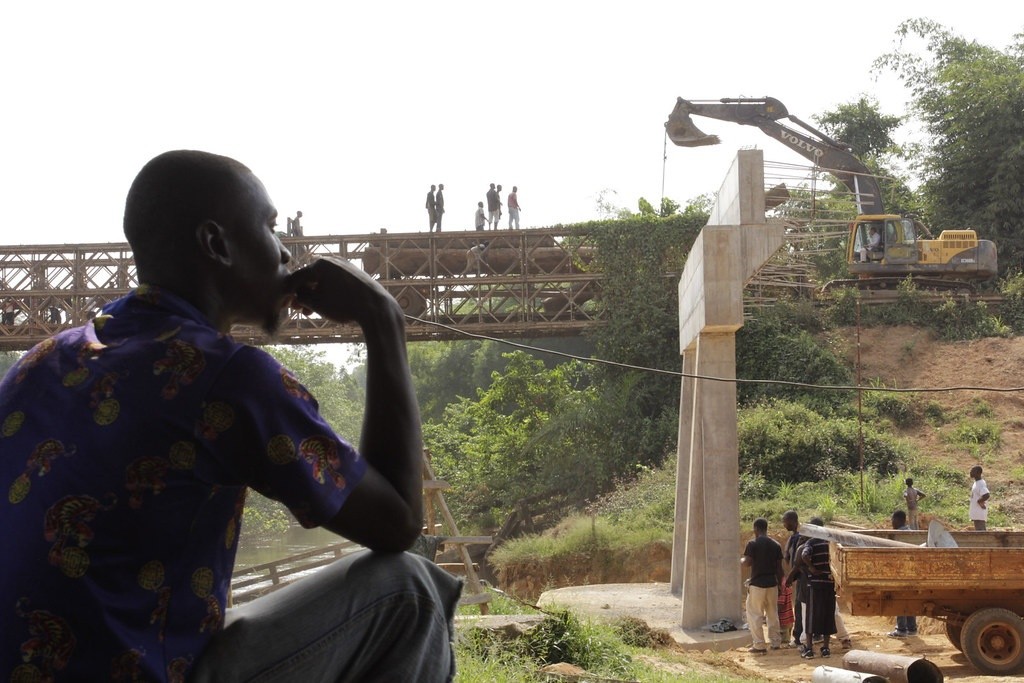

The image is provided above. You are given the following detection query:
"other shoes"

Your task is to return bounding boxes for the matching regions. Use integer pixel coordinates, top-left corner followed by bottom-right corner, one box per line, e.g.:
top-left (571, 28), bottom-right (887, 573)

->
top-left (781, 643), bottom-right (788, 650)
top-left (771, 645), bottom-right (778, 649)
top-left (888, 628), bottom-right (907, 638)
top-left (908, 629), bottom-right (917, 635)
top-left (748, 647), bottom-right (765, 653)
top-left (789, 640), bottom-right (803, 648)
top-left (820, 647), bottom-right (830, 658)
top-left (811, 634), bottom-right (824, 643)
top-left (801, 650), bottom-right (813, 659)
top-left (842, 640), bottom-right (850, 649)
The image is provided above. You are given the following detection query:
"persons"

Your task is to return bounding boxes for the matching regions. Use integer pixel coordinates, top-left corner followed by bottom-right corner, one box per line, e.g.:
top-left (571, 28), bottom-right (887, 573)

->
top-left (475, 202), bottom-right (488, 230)
top-left (903, 478), bottom-right (926, 530)
top-left (50, 307), bottom-right (61, 324)
top-left (859, 227), bottom-right (880, 262)
top-left (292, 211), bottom-right (302, 236)
top-left (1, 298), bottom-right (16, 325)
top-left (888, 509), bottom-right (918, 638)
top-left (463, 244), bottom-right (485, 278)
top-left (782, 510), bottom-right (852, 659)
top-left (969, 466), bottom-right (990, 531)
top-left (487, 183), bottom-right (503, 230)
top-left (426, 184), bottom-right (444, 232)
top-left (0, 149), bottom-right (463, 683)
top-left (85, 296), bottom-right (97, 320)
top-left (508, 186), bottom-right (521, 229)
top-left (742, 519), bottom-right (784, 652)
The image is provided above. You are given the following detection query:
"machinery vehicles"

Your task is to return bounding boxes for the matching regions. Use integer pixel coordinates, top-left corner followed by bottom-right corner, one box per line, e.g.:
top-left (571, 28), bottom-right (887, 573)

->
top-left (664, 96), bottom-right (999, 298)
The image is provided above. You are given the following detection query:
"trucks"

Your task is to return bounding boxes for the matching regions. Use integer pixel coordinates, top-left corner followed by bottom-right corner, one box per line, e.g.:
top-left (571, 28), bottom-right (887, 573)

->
top-left (829, 528), bottom-right (1024, 676)
